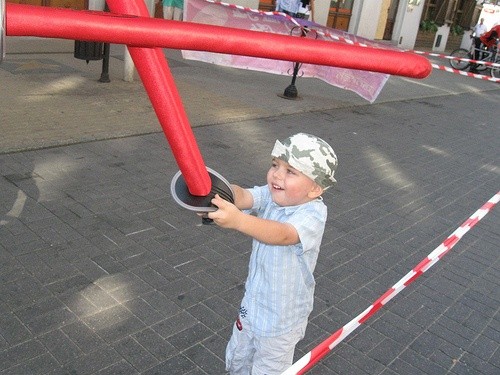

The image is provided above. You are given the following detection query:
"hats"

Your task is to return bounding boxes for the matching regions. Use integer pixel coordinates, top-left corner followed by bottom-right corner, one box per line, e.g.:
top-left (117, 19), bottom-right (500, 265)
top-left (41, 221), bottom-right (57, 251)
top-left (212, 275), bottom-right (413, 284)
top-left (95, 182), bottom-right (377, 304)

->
top-left (271, 133), bottom-right (338, 191)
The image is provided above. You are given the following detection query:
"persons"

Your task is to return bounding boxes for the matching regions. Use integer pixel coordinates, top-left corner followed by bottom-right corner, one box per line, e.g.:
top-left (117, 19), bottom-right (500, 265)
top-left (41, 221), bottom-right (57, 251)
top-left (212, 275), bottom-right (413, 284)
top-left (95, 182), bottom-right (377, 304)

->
top-left (471, 18), bottom-right (487, 60)
top-left (196, 132), bottom-right (339, 375)
top-left (274, 0), bottom-right (312, 21)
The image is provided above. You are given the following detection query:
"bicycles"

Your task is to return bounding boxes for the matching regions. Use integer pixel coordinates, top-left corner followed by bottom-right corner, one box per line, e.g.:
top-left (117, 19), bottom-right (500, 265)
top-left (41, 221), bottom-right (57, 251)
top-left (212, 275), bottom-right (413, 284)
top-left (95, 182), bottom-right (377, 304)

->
top-left (449, 33), bottom-right (500, 82)
